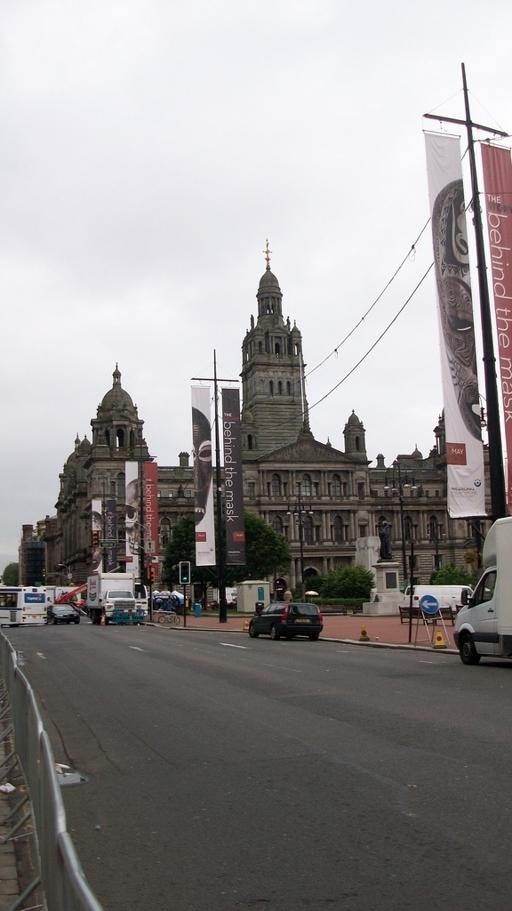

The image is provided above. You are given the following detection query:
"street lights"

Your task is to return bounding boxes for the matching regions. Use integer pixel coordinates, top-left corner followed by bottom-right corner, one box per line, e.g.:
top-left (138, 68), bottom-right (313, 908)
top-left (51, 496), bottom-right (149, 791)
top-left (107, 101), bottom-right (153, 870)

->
top-left (286, 497), bottom-right (314, 601)
top-left (57, 561), bottom-right (70, 585)
top-left (123, 519), bottom-right (154, 621)
top-left (382, 459), bottom-right (420, 589)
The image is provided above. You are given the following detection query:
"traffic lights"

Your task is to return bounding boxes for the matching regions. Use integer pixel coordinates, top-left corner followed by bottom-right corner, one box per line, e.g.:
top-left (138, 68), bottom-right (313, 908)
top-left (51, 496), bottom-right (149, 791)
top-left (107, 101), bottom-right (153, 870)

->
top-left (41, 567), bottom-right (45, 577)
top-left (178, 561), bottom-right (192, 585)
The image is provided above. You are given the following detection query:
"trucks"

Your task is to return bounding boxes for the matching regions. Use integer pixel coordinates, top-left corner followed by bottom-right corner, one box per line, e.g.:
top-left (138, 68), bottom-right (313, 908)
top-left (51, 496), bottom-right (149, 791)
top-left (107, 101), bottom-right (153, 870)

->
top-left (133, 581), bottom-right (149, 616)
top-left (453, 514), bottom-right (512, 667)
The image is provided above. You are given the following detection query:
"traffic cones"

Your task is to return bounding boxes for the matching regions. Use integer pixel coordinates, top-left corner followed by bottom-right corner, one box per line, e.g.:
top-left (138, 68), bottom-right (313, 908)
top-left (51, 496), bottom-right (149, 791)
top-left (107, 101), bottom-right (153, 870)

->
top-left (358, 622), bottom-right (371, 641)
top-left (100, 614), bottom-right (105, 625)
top-left (431, 627), bottom-right (448, 649)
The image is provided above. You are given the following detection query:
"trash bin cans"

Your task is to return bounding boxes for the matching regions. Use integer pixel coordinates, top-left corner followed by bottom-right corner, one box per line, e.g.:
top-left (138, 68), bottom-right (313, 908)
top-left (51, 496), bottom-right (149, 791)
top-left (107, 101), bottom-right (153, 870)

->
top-left (256, 602), bottom-right (264, 612)
top-left (195, 603), bottom-right (202, 616)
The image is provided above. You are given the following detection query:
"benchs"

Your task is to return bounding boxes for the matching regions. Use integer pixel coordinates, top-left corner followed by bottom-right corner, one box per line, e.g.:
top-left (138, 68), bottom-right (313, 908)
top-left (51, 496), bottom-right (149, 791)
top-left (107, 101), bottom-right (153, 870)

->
top-left (318, 605), bottom-right (347, 616)
top-left (351, 605), bottom-right (362, 614)
top-left (398, 605), bottom-right (454, 625)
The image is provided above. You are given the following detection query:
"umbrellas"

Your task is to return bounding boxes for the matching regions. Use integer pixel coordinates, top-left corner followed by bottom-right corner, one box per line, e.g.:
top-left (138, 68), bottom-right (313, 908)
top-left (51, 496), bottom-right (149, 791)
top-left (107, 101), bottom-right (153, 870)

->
top-left (305, 591), bottom-right (319, 603)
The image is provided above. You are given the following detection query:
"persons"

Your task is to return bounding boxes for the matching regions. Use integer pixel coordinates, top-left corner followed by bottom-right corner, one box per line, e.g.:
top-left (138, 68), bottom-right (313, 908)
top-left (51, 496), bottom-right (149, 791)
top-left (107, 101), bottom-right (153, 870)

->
top-left (284, 587), bottom-right (293, 602)
top-left (47, 597), bottom-right (54, 624)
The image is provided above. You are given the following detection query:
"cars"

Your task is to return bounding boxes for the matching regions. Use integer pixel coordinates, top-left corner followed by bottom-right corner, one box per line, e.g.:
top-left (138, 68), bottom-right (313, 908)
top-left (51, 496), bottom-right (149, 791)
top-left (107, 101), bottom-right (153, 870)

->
top-left (45, 602), bottom-right (83, 626)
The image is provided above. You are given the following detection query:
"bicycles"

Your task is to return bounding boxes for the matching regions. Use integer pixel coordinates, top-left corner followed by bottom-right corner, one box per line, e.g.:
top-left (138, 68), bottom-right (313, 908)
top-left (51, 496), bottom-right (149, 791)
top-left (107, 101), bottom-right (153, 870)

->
top-left (158, 610), bottom-right (181, 624)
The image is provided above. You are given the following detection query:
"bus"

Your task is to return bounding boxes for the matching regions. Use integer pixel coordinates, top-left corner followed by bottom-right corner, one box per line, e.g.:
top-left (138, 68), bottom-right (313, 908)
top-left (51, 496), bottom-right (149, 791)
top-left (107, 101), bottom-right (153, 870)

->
top-left (0, 584), bottom-right (50, 628)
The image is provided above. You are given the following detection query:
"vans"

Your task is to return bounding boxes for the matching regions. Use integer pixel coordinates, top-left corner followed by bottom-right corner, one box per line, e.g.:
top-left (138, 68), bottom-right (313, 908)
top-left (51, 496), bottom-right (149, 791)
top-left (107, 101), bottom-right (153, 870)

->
top-left (403, 582), bottom-right (491, 615)
top-left (246, 599), bottom-right (326, 642)
top-left (85, 571), bottom-right (140, 625)
